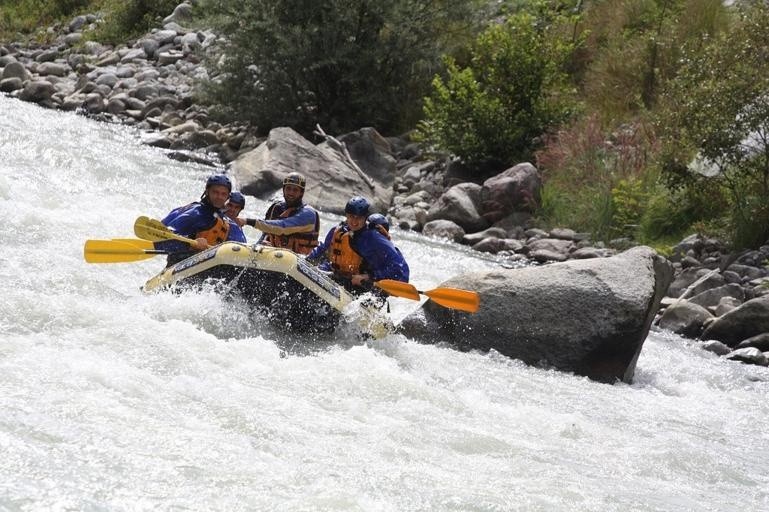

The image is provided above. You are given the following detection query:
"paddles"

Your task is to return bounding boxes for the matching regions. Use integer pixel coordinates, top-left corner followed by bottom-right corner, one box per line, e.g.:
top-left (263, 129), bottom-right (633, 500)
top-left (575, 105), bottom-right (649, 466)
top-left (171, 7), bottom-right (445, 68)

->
top-left (134, 216), bottom-right (212, 249)
top-left (329, 271), bottom-right (420, 303)
top-left (112, 237), bottom-right (156, 260)
top-left (83, 239), bottom-right (167, 263)
top-left (418, 288), bottom-right (480, 313)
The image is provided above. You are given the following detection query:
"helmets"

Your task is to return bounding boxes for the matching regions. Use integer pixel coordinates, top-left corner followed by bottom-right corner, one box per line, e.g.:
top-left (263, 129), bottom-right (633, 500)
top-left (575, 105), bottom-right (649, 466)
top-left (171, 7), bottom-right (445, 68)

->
top-left (205, 174), bottom-right (230, 192)
top-left (282, 172), bottom-right (306, 190)
top-left (368, 213), bottom-right (389, 233)
top-left (344, 194), bottom-right (369, 215)
top-left (228, 191), bottom-right (245, 209)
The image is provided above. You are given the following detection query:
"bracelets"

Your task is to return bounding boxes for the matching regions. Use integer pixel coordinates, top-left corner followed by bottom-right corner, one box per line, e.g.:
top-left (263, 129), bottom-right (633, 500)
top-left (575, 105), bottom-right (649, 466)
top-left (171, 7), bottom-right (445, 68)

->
top-left (247, 219), bottom-right (256, 227)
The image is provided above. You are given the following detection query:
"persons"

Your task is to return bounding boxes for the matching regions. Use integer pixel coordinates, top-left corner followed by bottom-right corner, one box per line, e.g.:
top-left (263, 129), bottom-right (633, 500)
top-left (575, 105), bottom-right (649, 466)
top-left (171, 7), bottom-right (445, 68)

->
top-left (164, 175), bottom-right (247, 266)
top-left (153, 192), bottom-right (244, 250)
top-left (369, 212), bottom-right (391, 236)
top-left (235, 172), bottom-right (321, 256)
top-left (305, 196), bottom-right (410, 302)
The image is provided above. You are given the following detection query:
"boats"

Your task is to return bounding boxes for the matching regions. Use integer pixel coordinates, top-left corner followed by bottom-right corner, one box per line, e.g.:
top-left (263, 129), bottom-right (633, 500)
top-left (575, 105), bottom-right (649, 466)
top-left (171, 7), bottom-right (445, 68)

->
top-left (141, 240), bottom-right (395, 343)
